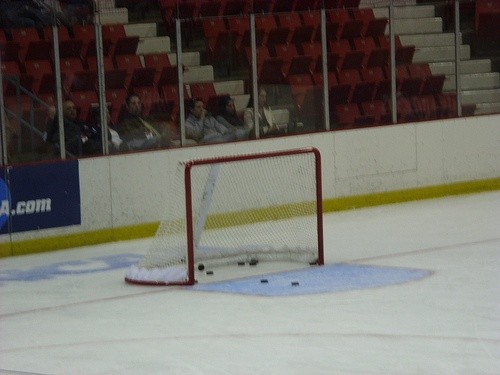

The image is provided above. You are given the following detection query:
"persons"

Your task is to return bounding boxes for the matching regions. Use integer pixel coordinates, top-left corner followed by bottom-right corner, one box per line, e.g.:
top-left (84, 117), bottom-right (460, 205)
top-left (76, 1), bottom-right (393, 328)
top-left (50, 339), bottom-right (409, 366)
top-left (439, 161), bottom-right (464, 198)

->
top-left (217, 98), bottom-right (264, 143)
top-left (52, 100), bottom-right (107, 160)
top-left (244, 87), bottom-right (283, 139)
top-left (120, 93), bottom-right (171, 151)
top-left (89, 107), bottom-right (130, 155)
top-left (181, 97), bottom-right (236, 148)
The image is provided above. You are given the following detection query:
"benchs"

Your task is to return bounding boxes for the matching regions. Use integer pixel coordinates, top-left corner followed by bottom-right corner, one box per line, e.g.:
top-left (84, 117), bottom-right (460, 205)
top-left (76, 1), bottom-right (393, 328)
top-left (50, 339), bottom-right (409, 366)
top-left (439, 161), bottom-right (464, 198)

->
top-left (0, 0), bottom-right (500, 167)
